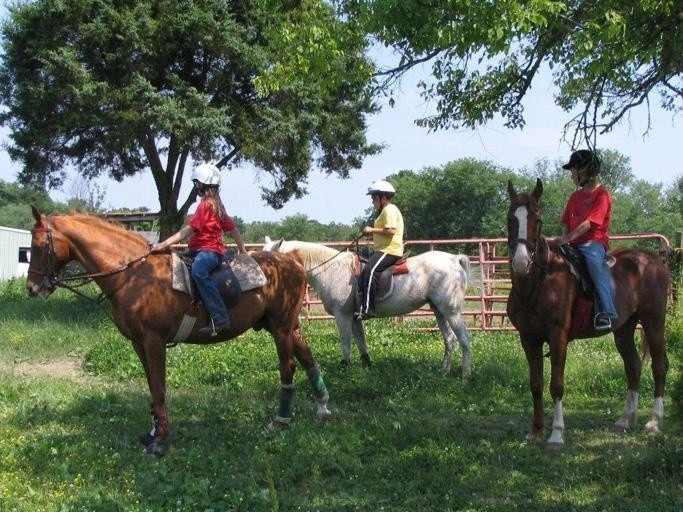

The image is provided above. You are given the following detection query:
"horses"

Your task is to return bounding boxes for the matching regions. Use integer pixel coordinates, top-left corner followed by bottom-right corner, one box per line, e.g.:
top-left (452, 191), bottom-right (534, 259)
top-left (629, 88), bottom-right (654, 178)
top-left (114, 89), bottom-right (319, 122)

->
top-left (261, 235), bottom-right (494, 385)
top-left (506, 177), bottom-right (675, 451)
top-left (26, 204), bottom-right (332, 460)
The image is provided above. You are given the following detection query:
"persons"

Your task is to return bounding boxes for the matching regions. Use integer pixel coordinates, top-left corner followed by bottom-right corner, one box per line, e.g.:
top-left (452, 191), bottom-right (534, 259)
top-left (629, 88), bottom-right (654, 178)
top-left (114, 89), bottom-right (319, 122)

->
top-left (353, 179), bottom-right (404, 320)
top-left (545, 149), bottom-right (618, 327)
top-left (149, 163), bottom-right (248, 336)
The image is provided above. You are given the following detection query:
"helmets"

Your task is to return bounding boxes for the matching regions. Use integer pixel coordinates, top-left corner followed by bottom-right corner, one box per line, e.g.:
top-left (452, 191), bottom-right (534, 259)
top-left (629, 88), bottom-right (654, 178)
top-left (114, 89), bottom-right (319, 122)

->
top-left (192, 164), bottom-right (221, 187)
top-left (366, 180), bottom-right (396, 198)
top-left (562, 150), bottom-right (600, 171)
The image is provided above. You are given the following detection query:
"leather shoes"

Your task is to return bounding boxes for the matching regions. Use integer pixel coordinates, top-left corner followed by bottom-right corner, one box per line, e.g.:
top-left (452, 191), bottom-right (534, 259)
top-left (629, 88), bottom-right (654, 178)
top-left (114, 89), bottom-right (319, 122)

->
top-left (353, 309), bottom-right (377, 319)
top-left (597, 317), bottom-right (616, 332)
top-left (200, 321), bottom-right (232, 339)
